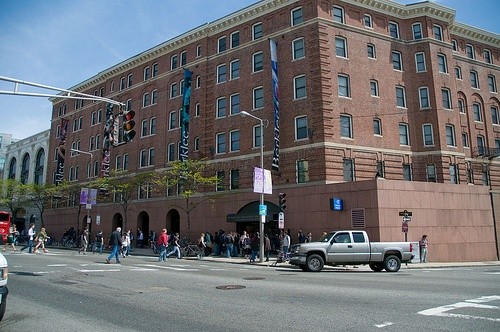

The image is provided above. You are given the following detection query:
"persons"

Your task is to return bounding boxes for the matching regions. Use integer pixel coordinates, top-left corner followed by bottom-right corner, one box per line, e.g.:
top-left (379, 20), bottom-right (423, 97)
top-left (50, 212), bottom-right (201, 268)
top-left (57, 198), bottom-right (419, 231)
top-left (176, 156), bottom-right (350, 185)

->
top-left (157, 229), bottom-right (168, 262)
top-left (104, 227), bottom-right (123, 265)
top-left (33, 228), bottom-right (50, 253)
top-left (298, 229), bottom-right (330, 243)
top-left (197, 230), bottom-right (279, 262)
top-left (20, 224), bottom-right (35, 253)
top-left (2, 223), bottom-right (18, 252)
top-left (166, 233), bottom-right (182, 259)
top-left (421, 235), bottom-right (428, 263)
top-left (121, 229), bottom-right (131, 256)
top-left (282, 231), bottom-right (290, 260)
top-left (79, 227), bottom-right (88, 255)
top-left (93, 231), bottom-right (103, 255)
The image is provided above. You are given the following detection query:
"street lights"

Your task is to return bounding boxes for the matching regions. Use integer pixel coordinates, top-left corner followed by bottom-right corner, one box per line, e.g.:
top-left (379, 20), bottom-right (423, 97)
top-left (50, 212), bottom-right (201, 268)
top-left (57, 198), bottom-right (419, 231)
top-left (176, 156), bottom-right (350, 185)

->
top-left (240, 110), bottom-right (264, 262)
top-left (70, 149), bottom-right (93, 243)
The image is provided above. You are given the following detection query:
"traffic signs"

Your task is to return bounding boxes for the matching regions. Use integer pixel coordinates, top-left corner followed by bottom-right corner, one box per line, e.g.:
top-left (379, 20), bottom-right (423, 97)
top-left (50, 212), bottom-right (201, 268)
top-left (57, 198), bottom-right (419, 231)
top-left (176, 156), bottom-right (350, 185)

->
top-left (399, 212), bottom-right (413, 221)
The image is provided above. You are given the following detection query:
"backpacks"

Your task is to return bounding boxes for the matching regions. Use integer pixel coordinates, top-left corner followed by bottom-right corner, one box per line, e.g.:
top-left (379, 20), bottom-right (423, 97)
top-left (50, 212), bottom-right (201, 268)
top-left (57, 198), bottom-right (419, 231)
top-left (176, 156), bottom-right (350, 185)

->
top-left (225, 236), bottom-right (232, 244)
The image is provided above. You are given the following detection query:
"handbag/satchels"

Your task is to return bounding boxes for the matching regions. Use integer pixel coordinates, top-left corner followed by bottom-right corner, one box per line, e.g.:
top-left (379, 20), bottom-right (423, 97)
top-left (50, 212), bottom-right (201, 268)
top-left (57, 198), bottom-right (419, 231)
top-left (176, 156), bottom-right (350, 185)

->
top-left (161, 241), bottom-right (168, 247)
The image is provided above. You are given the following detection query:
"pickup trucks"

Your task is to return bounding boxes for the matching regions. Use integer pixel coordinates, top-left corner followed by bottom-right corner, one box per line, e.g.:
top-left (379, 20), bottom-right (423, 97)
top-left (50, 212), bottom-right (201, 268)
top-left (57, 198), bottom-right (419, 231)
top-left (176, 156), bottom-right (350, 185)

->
top-left (289, 230), bottom-right (414, 272)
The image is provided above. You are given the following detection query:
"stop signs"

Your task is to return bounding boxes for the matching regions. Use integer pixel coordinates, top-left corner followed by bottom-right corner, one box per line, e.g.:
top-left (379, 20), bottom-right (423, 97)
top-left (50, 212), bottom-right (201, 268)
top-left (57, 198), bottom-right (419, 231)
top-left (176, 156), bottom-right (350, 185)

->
top-left (402, 224), bottom-right (408, 233)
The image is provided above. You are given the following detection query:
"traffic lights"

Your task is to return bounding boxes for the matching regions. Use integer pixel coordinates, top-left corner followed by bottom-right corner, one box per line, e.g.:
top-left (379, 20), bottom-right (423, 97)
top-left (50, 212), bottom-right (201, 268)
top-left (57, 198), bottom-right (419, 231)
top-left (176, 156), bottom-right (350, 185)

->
top-left (107, 115), bottom-right (119, 146)
top-left (279, 193), bottom-right (286, 211)
top-left (119, 110), bottom-right (136, 144)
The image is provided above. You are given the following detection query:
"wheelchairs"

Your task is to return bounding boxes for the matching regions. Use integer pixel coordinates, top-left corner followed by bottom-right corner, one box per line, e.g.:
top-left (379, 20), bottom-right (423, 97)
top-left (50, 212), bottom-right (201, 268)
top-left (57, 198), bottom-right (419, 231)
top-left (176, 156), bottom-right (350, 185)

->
top-left (59, 237), bottom-right (75, 248)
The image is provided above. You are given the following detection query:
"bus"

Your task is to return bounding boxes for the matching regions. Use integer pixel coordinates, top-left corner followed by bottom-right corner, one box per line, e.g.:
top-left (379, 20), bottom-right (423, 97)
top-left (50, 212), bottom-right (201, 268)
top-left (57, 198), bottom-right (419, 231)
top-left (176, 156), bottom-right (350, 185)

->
top-left (0, 211), bottom-right (10, 245)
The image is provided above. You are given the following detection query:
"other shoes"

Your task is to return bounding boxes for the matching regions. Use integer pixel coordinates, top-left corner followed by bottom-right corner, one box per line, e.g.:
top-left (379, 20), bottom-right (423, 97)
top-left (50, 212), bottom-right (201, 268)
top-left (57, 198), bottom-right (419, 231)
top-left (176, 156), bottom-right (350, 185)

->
top-left (106, 259), bottom-right (110, 264)
top-left (116, 261), bottom-right (121, 264)
